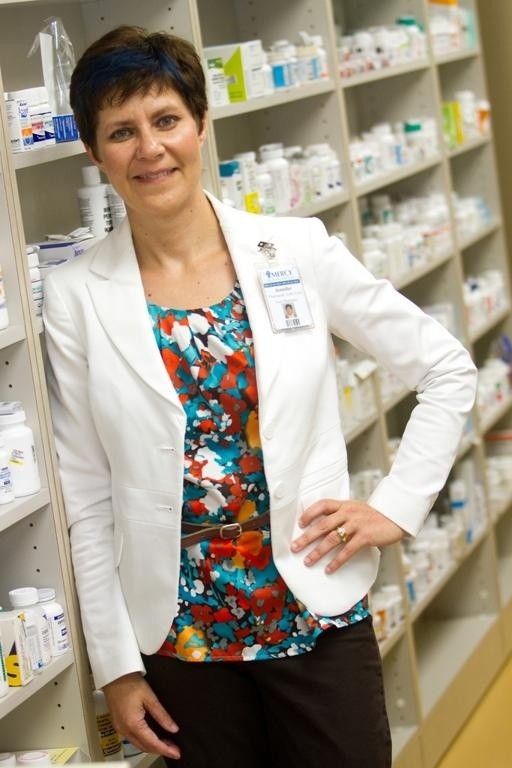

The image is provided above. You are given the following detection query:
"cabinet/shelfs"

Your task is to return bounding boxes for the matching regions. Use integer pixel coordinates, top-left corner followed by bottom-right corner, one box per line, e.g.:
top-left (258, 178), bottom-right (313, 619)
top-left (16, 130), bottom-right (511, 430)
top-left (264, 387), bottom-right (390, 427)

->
top-left (0, 0), bottom-right (512, 768)
top-left (1, 89), bottom-right (98, 767)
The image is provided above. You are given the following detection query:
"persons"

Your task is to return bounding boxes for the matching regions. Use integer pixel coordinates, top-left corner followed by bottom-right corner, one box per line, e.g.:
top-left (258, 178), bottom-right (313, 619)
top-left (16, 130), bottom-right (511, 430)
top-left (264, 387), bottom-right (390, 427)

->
top-left (285, 305), bottom-right (295, 318)
top-left (39, 24), bottom-right (479, 768)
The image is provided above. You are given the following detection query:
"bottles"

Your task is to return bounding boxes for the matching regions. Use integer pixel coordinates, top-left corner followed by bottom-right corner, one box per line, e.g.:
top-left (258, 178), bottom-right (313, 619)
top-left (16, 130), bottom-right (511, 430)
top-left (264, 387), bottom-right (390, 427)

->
top-left (268, 31), bottom-right (328, 91)
top-left (0, 266), bottom-right (10, 329)
top-left (4, 86), bottom-right (56, 154)
top-left (335, 16), bottom-right (428, 80)
top-left (220, 143), bottom-right (340, 218)
top-left (348, 118), bottom-right (439, 185)
top-left (0, 410), bottom-right (42, 497)
top-left (89, 672), bottom-right (143, 761)
top-left (36, 588), bottom-right (70, 658)
top-left (77, 165), bottom-right (111, 240)
top-left (0, 444), bottom-right (13, 506)
top-left (8, 587), bottom-right (52, 667)
top-left (370, 582), bottom-right (405, 641)
top-left (454, 89), bottom-right (478, 140)
top-left (356, 190), bottom-right (451, 283)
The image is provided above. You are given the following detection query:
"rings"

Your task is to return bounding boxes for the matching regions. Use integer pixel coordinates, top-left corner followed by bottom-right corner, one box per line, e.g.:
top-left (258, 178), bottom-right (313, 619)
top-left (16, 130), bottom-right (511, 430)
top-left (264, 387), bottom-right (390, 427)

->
top-left (336, 526), bottom-right (349, 541)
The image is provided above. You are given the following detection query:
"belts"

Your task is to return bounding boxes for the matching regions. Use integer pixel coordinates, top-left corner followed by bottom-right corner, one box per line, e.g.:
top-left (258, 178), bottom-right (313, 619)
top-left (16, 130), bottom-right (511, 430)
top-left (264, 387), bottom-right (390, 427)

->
top-left (181, 510), bottom-right (270, 551)
